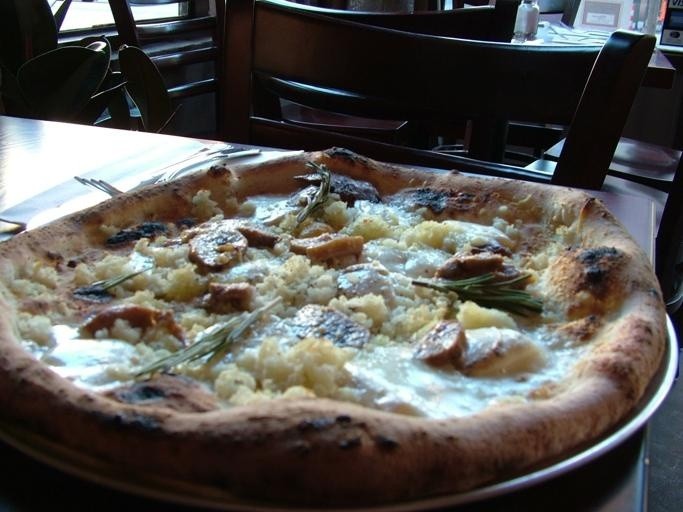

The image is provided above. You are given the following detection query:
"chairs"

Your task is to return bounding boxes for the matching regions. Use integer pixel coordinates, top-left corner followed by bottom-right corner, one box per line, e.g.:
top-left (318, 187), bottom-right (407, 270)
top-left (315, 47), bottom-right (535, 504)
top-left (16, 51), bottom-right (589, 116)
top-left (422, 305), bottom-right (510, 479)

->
top-left (218, 2), bottom-right (657, 193)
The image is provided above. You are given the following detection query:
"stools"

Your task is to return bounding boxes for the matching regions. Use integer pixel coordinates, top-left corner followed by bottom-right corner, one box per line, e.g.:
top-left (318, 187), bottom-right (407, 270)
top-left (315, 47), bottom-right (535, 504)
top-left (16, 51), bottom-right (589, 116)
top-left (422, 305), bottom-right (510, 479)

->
top-left (521, 128), bottom-right (682, 243)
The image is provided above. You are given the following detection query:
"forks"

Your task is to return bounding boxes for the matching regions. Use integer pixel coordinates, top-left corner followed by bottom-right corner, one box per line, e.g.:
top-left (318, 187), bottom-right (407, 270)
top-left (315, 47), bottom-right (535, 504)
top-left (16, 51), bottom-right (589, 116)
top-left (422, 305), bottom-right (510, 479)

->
top-left (549, 21), bottom-right (610, 43)
top-left (73, 145), bottom-right (262, 198)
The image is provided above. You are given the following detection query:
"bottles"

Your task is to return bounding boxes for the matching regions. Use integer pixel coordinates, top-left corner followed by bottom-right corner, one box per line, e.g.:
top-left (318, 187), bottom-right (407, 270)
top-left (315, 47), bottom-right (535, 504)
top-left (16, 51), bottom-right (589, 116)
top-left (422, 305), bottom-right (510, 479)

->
top-left (513, 0), bottom-right (539, 43)
